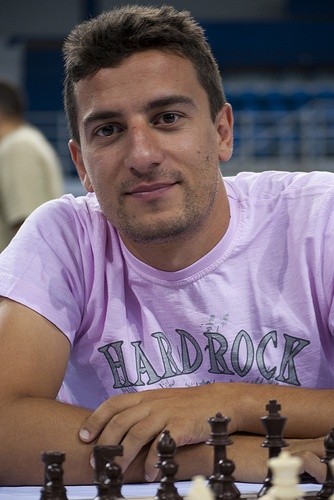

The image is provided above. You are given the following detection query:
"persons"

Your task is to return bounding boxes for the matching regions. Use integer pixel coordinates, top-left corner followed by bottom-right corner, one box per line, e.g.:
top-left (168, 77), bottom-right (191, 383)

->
top-left (0, 5), bottom-right (334, 484)
top-left (0, 81), bottom-right (61, 251)
